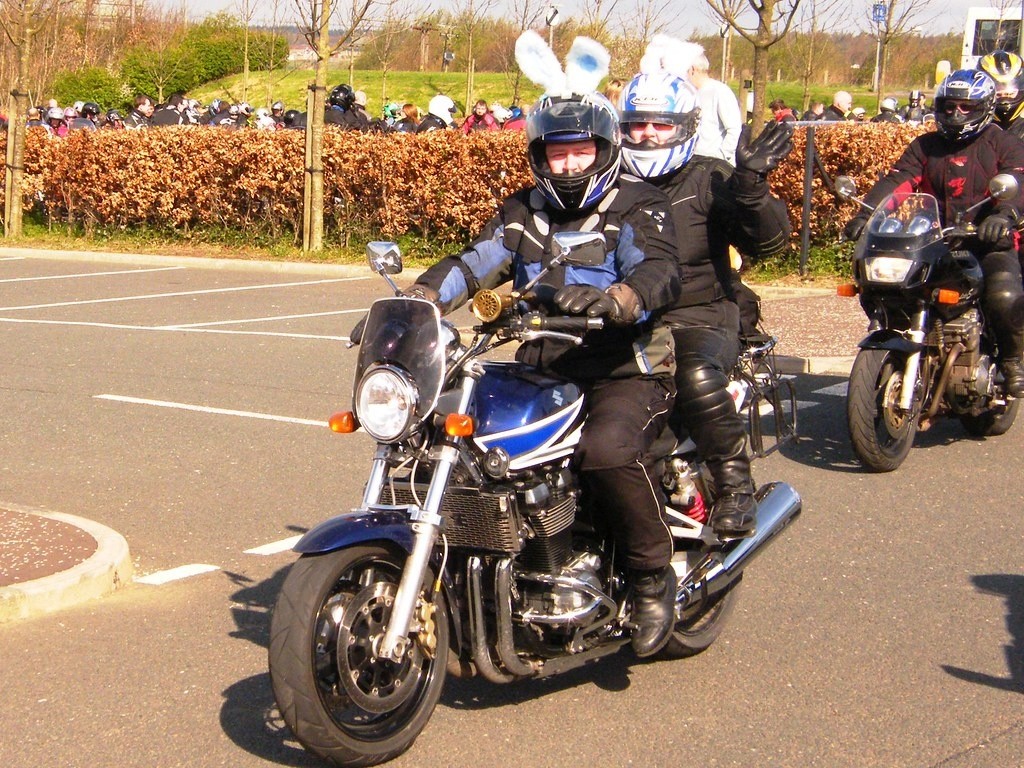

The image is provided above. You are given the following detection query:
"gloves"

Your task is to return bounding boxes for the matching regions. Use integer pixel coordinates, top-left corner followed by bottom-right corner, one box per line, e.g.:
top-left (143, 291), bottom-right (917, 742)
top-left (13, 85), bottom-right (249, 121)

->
top-left (350, 284), bottom-right (444, 345)
top-left (977, 208), bottom-right (1017, 244)
top-left (734, 119), bottom-right (795, 183)
top-left (844, 218), bottom-right (868, 241)
top-left (555, 282), bottom-right (643, 326)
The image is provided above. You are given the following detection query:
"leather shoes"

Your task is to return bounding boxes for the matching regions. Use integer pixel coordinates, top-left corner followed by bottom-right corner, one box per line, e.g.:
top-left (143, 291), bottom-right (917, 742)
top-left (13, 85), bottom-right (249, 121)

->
top-left (1001, 359), bottom-right (1024, 398)
top-left (629, 561), bottom-right (677, 656)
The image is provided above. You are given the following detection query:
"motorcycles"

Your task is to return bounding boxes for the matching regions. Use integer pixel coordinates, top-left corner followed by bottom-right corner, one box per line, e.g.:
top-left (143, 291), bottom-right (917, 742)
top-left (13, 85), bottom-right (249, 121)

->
top-left (834, 173), bottom-right (1024, 472)
top-left (267, 241), bottom-right (802, 768)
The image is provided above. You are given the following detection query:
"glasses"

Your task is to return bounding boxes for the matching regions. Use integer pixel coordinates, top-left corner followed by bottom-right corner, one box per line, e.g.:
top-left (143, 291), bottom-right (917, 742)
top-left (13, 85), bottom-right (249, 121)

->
top-left (944, 102), bottom-right (977, 112)
top-left (629, 118), bottom-right (675, 131)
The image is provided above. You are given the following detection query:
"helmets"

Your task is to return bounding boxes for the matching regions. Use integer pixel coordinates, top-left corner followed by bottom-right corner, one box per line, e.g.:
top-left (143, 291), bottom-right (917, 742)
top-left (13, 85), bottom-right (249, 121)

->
top-left (976, 51), bottom-right (1024, 128)
top-left (617, 71), bottom-right (702, 178)
top-left (852, 107), bottom-right (866, 120)
top-left (524, 83), bottom-right (621, 212)
top-left (935, 69), bottom-right (996, 141)
top-left (508, 106), bottom-right (521, 119)
top-left (329, 84), bottom-right (424, 121)
top-left (46, 99), bottom-right (125, 128)
top-left (429, 95), bottom-right (457, 128)
top-left (184, 96), bottom-right (302, 126)
top-left (880, 97), bottom-right (898, 114)
top-left (909, 90), bottom-right (921, 108)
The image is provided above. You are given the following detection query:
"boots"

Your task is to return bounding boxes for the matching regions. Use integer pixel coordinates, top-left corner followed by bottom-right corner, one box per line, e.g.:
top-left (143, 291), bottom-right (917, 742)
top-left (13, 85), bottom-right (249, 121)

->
top-left (688, 410), bottom-right (757, 532)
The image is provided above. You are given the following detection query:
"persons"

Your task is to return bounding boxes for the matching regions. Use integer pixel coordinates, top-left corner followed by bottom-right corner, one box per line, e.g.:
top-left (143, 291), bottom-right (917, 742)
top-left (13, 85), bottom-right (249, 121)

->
top-left (975, 51), bottom-right (1024, 141)
top-left (352, 91), bottom-right (682, 657)
top-left (615, 70), bottom-right (795, 530)
top-left (324, 84), bottom-right (527, 137)
top-left (687, 53), bottom-right (741, 168)
top-left (846, 69), bottom-right (1024, 399)
top-left (769, 90), bottom-right (930, 123)
top-left (28, 94), bottom-right (308, 139)
